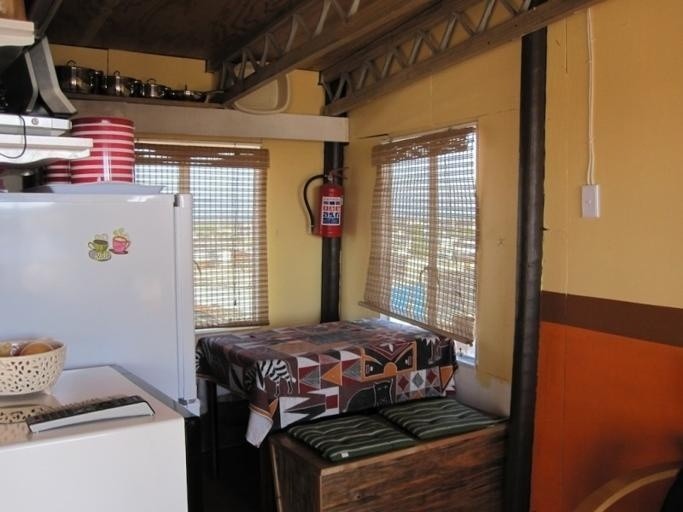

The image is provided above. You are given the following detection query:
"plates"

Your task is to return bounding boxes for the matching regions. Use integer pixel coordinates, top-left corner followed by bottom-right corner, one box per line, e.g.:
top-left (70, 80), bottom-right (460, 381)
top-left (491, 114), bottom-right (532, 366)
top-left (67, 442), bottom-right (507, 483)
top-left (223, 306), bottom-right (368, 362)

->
top-left (46, 116), bottom-right (136, 185)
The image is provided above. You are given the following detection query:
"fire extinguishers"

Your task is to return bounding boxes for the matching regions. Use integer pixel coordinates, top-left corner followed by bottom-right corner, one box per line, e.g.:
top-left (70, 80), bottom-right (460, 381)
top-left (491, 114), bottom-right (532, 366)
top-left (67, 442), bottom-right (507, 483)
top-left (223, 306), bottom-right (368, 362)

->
top-left (304, 167), bottom-right (349, 238)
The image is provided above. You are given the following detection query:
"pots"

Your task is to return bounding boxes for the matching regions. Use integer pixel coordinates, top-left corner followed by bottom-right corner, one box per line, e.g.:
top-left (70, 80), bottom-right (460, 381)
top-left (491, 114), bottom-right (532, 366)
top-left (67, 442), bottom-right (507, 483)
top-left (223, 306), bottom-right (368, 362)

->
top-left (103, 70), bottom-right (142, 97)
top-left (55, 59), bottom-right (103, 95)
top-left (167, 84), bottom-right (224, 103)
top-left (140, 77), bottom-right (171, 99)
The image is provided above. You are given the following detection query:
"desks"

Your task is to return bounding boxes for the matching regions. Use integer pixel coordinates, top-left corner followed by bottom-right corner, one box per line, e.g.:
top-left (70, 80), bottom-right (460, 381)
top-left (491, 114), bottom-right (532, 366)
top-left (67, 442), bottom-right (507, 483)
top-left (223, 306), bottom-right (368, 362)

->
top-left (195, 319), bottom-right (457, 512)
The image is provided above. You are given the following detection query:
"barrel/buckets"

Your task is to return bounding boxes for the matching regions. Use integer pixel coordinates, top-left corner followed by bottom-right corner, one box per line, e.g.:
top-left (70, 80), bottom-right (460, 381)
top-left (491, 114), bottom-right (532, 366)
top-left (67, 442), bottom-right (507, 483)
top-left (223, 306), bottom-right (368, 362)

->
top-left (48, 158), bottom-right (69, 183)
top-left (71, 116), bottom-right (136, 182)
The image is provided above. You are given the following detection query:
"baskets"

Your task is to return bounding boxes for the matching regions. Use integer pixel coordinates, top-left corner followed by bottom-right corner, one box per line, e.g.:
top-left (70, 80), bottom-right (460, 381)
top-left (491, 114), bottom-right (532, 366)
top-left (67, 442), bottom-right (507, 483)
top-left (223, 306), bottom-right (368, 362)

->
top-left (0, 338), bottom-right (65, 397)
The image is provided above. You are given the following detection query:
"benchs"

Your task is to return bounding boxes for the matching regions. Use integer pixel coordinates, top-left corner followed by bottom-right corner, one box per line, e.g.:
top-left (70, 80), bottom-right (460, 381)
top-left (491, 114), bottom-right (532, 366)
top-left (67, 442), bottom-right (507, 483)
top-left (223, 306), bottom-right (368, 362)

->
top-left (268, 399), bottom-right (510, 511)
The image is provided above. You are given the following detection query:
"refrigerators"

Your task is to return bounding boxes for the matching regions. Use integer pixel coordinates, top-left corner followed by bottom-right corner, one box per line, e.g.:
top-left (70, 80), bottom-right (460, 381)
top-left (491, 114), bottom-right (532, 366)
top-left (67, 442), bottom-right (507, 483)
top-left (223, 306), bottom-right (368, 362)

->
top-left (0, 191), bottom-right (203, 420)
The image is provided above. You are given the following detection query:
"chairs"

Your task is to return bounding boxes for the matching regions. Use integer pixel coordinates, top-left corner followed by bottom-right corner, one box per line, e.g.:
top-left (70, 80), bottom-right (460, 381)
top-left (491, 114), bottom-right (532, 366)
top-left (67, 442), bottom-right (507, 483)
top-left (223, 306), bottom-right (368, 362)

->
top-left (575, 464), bottom-right (681, 512)
